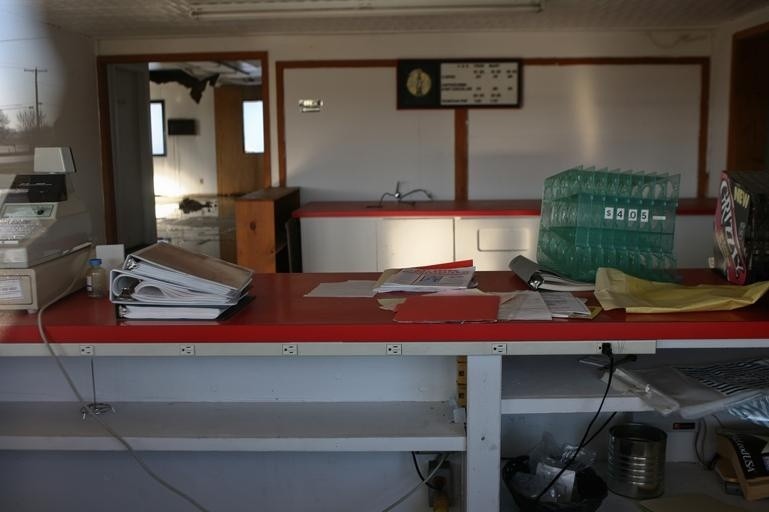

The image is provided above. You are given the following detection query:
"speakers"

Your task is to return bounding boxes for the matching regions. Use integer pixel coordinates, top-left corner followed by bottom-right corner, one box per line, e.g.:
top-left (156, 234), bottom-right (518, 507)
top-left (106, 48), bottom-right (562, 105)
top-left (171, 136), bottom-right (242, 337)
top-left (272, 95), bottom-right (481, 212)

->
top-left (168, 118), bottom-right (197, 136)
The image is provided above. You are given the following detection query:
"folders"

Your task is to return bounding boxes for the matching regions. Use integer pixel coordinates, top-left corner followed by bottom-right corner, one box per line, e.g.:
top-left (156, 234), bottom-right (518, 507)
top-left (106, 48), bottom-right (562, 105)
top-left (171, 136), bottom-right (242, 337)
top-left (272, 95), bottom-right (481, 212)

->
top-left (109, 241), bottom-right (256, 322)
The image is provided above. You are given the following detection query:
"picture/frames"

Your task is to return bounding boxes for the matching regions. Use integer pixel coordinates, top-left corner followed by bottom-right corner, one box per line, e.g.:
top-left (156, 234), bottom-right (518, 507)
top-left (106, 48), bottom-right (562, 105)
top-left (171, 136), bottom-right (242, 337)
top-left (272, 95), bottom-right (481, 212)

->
top-left (441, 58), bottom-right (524, 109)
top-left (398, 61), bottom-right (441, 109)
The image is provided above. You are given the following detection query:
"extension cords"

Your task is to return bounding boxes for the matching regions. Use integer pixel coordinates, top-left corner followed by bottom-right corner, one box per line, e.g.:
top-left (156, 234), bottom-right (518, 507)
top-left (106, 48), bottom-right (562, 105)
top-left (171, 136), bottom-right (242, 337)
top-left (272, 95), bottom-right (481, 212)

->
top-left (429, 459), bottom-right (454, 507)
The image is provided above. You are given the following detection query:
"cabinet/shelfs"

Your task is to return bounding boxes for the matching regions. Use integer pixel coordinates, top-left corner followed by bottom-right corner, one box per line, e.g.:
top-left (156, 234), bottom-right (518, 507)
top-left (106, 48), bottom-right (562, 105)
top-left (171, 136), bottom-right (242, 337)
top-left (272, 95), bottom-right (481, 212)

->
top-left (457, 219), bottom-right (540, 270)
top-left (299, 219), bottom-right (456, 274)
top-left (234, 186), bottom-right (301, 274)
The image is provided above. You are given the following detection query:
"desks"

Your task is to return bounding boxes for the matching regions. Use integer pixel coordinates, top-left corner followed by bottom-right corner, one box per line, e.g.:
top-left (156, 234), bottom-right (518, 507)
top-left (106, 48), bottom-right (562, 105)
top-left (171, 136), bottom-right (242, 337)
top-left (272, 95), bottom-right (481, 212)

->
top-left (1, 271), bottom-right (769, 511)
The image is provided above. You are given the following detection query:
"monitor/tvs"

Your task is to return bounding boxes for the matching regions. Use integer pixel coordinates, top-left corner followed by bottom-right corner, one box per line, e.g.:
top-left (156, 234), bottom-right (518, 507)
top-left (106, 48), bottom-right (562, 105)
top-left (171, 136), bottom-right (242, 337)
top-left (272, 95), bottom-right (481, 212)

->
top-left (150, 101), bottom-right (166, 157)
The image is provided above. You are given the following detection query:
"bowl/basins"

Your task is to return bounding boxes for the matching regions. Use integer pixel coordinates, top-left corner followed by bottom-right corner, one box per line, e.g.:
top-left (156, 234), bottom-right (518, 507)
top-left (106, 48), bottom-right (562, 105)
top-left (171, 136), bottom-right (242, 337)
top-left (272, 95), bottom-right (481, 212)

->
top-left (504, 456), bottom-right (608, 511)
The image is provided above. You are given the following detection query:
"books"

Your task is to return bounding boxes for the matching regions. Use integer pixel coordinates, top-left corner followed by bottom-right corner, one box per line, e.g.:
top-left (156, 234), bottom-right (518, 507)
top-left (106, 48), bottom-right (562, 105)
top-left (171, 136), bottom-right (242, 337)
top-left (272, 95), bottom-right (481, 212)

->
top-left (369, 257), bottom-right (477, 291)
top-left (711, 422), bottom-right (769, 503)
top-left (107, 246), bottom-right (249, 322)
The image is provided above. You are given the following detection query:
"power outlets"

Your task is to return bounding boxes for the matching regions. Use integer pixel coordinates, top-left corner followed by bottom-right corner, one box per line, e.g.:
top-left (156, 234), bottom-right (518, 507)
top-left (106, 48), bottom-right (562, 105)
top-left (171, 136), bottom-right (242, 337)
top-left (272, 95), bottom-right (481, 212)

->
top-left (283, 343), bottom-right (298, 358)
top-left (492, 342), bottom-right (508, 355)
top-left (78, 344), bottom-right (95, 356)
top-left (594, 342), bottom-right (612, 356)
top-left (179, 344), bottom-right (195, 358)
top-left (387, 343), bottom-right (402, 356)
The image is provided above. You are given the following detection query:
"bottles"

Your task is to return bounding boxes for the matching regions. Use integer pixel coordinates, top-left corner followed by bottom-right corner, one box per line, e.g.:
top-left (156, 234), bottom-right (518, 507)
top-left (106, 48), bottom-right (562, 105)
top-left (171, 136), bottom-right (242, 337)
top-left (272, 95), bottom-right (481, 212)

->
top-left (86, 260), bottom-right (107, 298)
